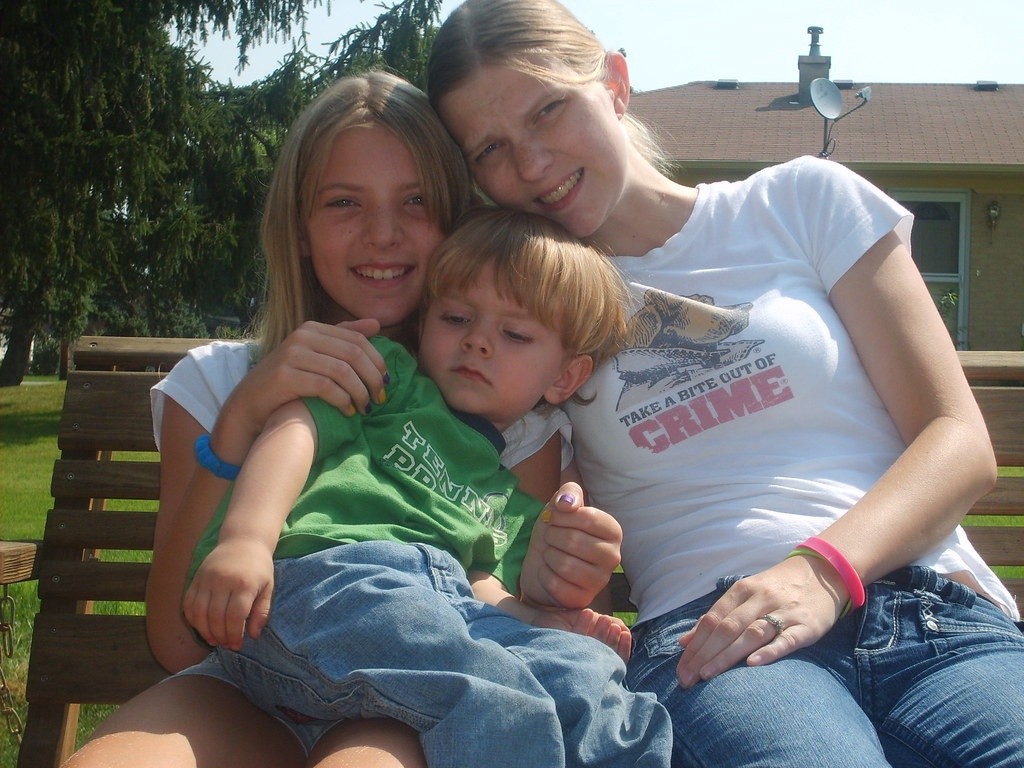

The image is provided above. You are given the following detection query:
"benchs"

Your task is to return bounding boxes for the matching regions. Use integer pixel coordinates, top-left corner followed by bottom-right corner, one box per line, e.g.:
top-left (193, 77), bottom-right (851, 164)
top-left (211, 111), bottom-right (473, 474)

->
top-left (16, 337), bottom-right (1024, 768)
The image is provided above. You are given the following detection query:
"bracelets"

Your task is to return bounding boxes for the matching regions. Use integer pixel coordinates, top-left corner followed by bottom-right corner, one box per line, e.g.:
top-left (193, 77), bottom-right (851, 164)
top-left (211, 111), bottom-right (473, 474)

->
top-left (783, 537), bottom-right (864, 618)
top-left (194, 435), bottom-right (240, 481)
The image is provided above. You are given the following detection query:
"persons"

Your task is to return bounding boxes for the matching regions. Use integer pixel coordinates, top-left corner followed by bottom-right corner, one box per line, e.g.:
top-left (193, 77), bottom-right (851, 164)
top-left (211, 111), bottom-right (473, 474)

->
top-left (59, 73), bottom-right (620, 768)
top-left (431, 0), bottom-right (1024, 768)
top-left (181, 206), bottom-right (673, 768)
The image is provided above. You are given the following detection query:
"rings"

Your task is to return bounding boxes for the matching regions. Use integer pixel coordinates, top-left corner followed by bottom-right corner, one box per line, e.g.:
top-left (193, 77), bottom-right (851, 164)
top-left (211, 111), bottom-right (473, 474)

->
top-left (757, 614), bottom-right (784, 635)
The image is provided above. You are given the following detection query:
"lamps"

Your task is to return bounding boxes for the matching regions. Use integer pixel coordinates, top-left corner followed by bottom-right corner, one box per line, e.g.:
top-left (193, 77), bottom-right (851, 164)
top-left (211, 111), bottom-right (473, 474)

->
top-left (987, 201), bottom-right (1000, 243)
